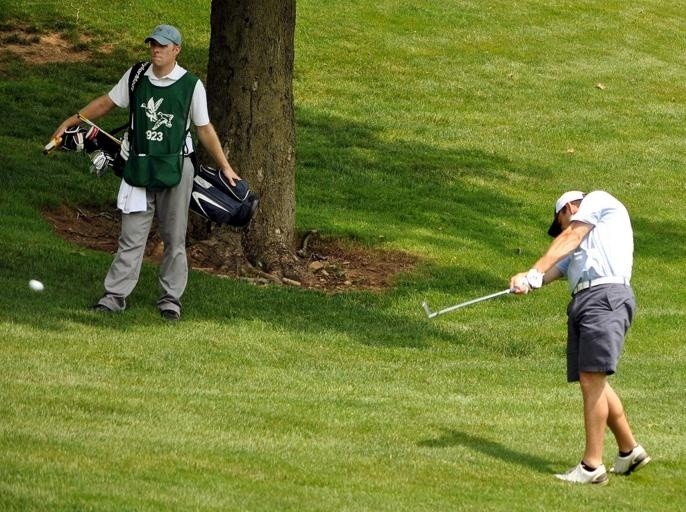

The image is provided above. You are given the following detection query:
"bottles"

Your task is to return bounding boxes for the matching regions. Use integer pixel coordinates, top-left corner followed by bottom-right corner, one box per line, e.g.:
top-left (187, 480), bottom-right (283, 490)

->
top-left (41, 137), bottom-right (62, 156)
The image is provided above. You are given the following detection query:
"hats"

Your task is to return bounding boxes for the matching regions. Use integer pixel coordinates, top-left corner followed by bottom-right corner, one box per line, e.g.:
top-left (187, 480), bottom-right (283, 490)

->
top-left (144, 24), bottom-right (181, 46)
top-left (548, 191), bottom-right (587, 238)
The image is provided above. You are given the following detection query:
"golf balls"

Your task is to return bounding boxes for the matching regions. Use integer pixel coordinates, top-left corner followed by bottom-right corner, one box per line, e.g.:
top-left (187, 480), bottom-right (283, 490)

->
top-left (30, 280), bottom-right (43, 292)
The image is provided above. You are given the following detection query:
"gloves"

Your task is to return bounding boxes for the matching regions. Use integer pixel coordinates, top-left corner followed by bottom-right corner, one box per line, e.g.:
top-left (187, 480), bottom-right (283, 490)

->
top-left (510, 268), bottom-right (546, 294)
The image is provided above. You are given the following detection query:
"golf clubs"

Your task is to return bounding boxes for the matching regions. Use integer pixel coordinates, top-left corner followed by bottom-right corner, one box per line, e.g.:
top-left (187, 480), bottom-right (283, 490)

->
top-left (422, 287), bottom-right (517, 319)
top-left (43, 124), bottom-right (116, 179)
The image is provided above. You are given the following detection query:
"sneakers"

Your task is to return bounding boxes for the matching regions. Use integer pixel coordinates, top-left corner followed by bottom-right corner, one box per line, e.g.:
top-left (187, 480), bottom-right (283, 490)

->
top-left (90, 294), bottom-right (180, 321)
top-left (606, 444), bottom-right (652, 476)
top-left (552, 461), bottom-right (609, 489)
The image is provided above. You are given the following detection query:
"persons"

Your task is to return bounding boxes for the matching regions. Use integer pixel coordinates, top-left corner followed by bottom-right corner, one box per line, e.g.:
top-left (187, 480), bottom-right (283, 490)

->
top-left (508, 187), bottom-right (653, 487)
top-left (51, 24), bottom-right (241, 323)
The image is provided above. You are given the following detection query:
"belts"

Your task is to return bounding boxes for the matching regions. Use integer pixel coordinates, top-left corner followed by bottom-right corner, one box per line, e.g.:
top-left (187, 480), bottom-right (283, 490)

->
top-left (573, 276), bottom-right (630, 295)
